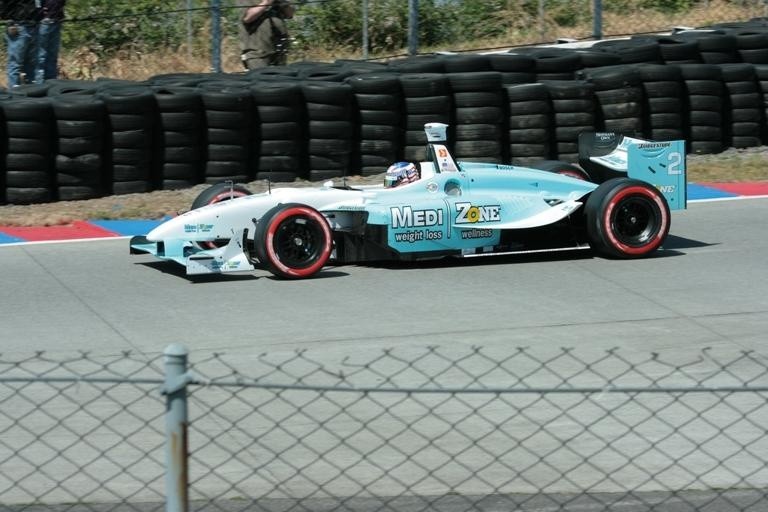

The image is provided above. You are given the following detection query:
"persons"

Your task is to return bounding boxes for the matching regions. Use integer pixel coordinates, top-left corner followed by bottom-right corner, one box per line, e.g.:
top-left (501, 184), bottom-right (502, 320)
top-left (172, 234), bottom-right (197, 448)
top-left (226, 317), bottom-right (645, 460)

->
top-left (238, 0), bottom-right (294, 72)
top-left (0, 0), bottom-right (65, 88)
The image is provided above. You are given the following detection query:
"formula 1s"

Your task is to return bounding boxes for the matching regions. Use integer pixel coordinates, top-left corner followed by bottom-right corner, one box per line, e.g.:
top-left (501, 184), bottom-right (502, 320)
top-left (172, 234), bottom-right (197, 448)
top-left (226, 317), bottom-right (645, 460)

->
top-left (129, 120), bottom-right (686, 278)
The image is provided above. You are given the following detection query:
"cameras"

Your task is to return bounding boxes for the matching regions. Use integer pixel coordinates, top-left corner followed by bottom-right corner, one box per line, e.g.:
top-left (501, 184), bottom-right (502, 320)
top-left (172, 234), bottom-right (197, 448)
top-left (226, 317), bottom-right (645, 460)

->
top-left (275, 37), bottom-right (289, 56)
